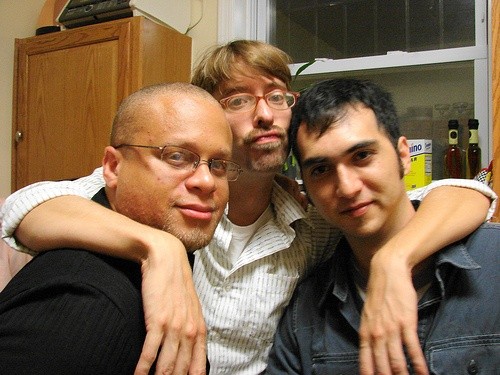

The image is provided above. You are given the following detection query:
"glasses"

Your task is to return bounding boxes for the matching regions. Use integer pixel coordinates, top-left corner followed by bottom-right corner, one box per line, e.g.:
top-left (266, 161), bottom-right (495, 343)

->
top-left (115, 144), bottom-right (244, 181)
top-left (219, 90), bottom-right (300, 112)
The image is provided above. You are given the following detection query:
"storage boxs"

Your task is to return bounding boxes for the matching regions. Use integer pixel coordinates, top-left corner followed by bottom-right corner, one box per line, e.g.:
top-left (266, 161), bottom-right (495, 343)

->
top-left (403, 138), bottom-right (432, 191)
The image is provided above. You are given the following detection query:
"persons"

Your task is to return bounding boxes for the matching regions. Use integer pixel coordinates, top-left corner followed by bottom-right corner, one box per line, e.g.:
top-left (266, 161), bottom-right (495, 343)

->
top-left (0, 82), bottom-right (234, 375)
top-left (1, 40), bottom-right (498, 375)
top-left (268, 78), bottom-right (500, 375)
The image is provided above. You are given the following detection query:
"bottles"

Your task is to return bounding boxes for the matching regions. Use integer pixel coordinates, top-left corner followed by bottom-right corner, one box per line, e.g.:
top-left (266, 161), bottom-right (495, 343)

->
top-left (439, 118), bottom-right (464, 179)
top-left (464, 119), bottom-right (481, 179)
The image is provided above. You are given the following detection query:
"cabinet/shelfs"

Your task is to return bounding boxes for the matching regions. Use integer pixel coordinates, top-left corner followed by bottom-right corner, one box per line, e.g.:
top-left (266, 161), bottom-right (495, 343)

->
top-left (10, 16), bottom-right (193, 191)
